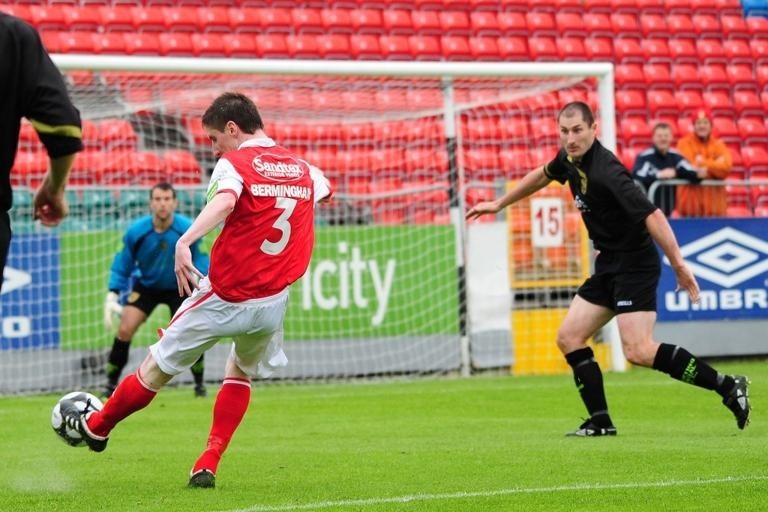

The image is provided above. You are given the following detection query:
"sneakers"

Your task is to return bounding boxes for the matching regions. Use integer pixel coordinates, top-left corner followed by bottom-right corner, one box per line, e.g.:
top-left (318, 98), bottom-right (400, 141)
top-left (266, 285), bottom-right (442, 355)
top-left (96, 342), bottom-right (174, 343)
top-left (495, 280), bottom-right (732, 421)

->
top-left (722, 375), bottom-right (754, 430)
top-left (186, 466), bottom-right (216, 489)
top-left (59, 397), bottom-right (109, 453)
top-left (564, 417), bottom-right (617, 437)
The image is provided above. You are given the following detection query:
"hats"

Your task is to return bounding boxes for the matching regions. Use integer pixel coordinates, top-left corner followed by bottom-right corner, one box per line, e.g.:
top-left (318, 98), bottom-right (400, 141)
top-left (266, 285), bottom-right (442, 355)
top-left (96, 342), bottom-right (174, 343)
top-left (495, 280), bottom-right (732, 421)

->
top-left (691, 108), bottom-right (714, 127)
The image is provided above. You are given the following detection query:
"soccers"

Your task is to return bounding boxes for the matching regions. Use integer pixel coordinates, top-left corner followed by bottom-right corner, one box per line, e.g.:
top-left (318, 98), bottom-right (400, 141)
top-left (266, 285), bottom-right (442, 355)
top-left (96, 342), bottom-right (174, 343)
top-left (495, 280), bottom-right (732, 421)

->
top-left (52, 392), bottom-right (104, 447)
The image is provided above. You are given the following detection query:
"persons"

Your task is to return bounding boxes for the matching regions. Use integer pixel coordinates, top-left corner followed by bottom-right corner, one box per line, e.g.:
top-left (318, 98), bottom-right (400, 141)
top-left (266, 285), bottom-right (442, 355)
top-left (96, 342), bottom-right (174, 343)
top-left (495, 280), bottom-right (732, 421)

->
top-left (630, 120), bottom-right (704, 219)
top-left (58, 91), bottom-right (335, 492)
top-left (100, 181), bottom-right (209, 402)
top-left (466, 100), bottom-right (755, 435)
top-left (675, 110), bottom-right (735, 219)
top-left (0, 11), bottom-right (85, 294)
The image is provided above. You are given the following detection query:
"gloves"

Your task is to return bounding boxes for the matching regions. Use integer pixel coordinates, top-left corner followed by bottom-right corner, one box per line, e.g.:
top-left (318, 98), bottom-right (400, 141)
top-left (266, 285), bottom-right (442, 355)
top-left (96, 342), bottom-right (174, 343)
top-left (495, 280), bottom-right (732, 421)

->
top-left (103, 291), bottom-right (124, 330)
